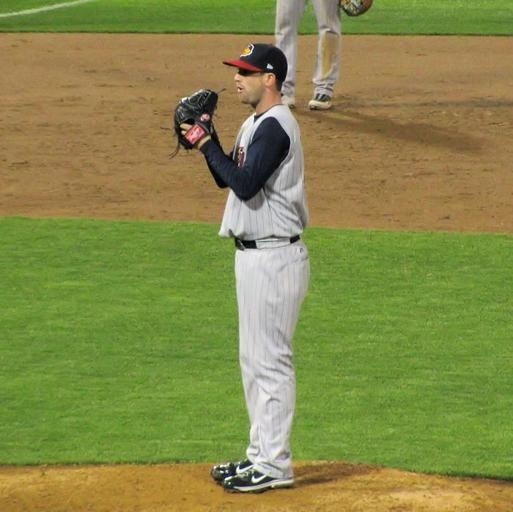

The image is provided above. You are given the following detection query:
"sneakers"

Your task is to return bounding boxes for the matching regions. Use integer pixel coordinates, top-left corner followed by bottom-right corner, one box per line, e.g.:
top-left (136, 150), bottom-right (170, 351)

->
top-left (306, 91), bottom-right (335, 110)
top-left (209, 455), bottom-right (253, 483)
top-left (280, 93), bottom-right (296, 110)
top-left (221, 463), bottom-right (296, 494)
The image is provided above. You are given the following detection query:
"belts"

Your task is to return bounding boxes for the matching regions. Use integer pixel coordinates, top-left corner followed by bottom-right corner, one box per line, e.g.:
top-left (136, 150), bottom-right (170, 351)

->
top-left (233, 235), bottom-right (303, 251)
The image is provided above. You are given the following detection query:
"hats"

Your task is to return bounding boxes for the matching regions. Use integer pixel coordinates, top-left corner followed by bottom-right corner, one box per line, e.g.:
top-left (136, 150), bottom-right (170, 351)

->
top-left (220, 41), bottom-right (289, 84)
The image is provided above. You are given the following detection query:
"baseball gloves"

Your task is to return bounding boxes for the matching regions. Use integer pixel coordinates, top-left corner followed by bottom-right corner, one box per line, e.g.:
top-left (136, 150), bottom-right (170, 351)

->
top-left (173, 90), bottom-right (218, 150)
top-left (340, 1), bottom-right (372, 16)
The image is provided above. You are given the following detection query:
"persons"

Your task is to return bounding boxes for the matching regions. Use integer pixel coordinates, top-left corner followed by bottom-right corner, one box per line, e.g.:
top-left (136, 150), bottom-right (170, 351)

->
top-left (271, 0), bottom-right (343, 111)
top-left (176, 43), bottom-right (311, 495)
